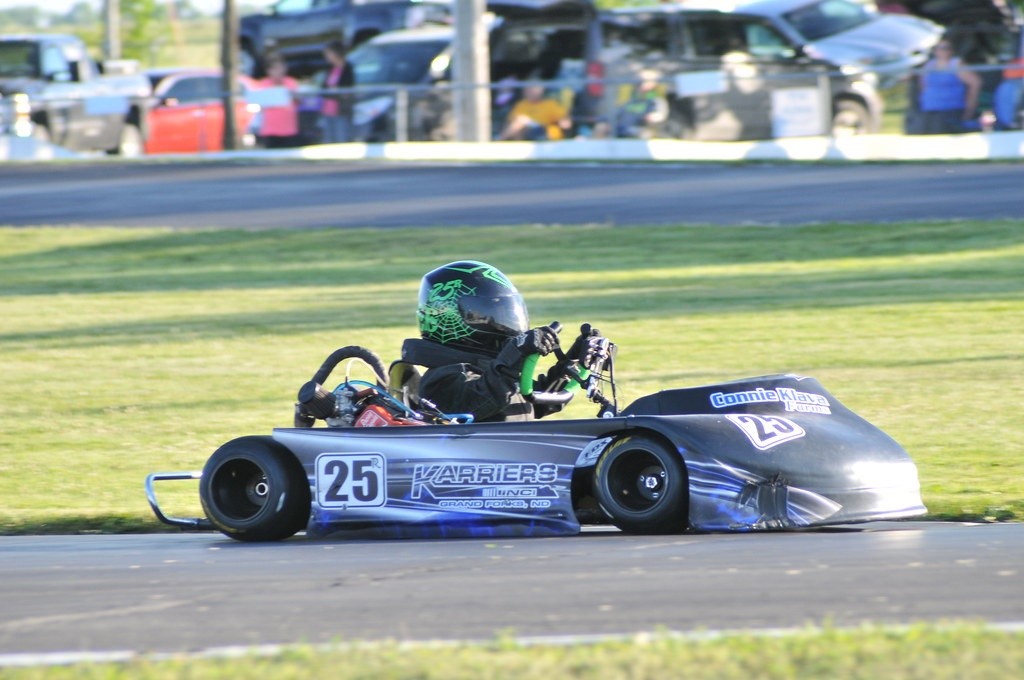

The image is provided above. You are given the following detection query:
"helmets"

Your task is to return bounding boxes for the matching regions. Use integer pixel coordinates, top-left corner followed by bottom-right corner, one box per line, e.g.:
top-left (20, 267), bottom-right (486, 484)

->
top-left (416, 259), bottom-right (531, 357)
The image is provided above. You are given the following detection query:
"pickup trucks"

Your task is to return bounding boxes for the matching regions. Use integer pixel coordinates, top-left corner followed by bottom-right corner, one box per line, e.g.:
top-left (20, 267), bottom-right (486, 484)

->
top-left (1, 36), bottom-right (155, 156)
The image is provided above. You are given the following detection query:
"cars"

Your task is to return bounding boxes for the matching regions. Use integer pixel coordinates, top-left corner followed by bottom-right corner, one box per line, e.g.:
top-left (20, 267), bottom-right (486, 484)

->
top-left (727, 2), bottom-right (949, 94)
top-left (301, 25), bottom-right (460, 142)
top-left (487, 0), bottom-right (614, 83)
top-left (131, 69), bottom-right (258, 155)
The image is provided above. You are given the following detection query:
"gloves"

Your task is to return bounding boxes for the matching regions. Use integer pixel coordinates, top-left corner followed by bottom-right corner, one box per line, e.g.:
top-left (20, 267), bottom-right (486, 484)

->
top-left (512, 324), bottom-right (559, 357)
top-left (565, 328), bottom-right (600, 359)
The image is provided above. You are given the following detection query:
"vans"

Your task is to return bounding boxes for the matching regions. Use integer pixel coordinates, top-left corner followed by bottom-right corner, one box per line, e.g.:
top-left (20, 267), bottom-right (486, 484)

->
top-left (586, 6), bottom-right (883, 136)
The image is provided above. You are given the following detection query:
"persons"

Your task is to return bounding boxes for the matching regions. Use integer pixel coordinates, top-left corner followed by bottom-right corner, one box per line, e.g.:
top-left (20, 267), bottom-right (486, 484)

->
top-left (253, 48), bottom-right (304, 148)
top-left (316, 41), bottom-right (357, 148)
top-left (911, 38), bottom-right (982, 135)
top-left (496, 77), bottom-right (572, 141)
top-left (416, 259), bottom-right (614, 422)
top-left (572, 79), bottom-right (670, 142)
